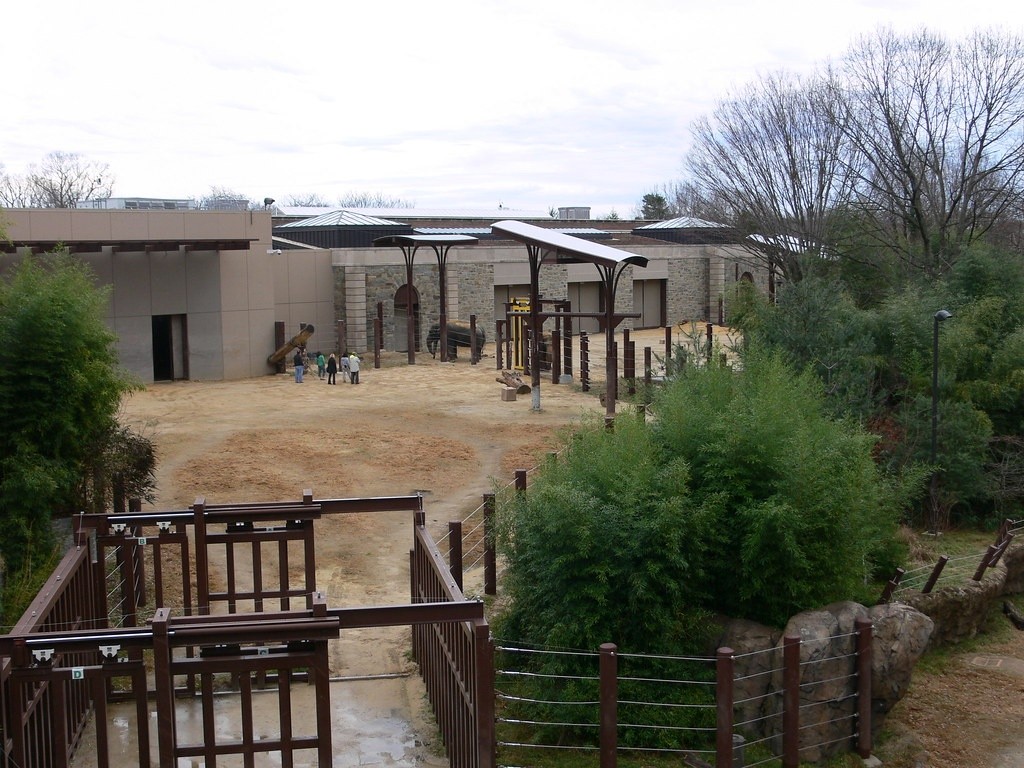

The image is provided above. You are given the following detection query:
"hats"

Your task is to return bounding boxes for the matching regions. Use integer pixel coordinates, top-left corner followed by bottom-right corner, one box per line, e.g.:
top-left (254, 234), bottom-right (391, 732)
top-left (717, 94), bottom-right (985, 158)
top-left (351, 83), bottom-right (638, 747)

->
top-left (330, 354), bottom-right (335, 357)
top-left (352, 352), bottom-right (356, 354)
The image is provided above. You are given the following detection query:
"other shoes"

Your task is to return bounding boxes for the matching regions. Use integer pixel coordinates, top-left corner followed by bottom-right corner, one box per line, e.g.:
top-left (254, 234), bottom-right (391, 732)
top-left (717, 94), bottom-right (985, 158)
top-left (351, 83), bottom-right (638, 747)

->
top-left (295, 382), bottom-right (304, 383)
top-left (333, 384), bottom-right (336, 385)
top-left (320, 378), bottom-right (326, 380)
top-left (343, 380), bottom-right (346, 383)
top-left (328, 383), bottom-right (332, 385)
top-left (351, 383), bottom-right (360, 384)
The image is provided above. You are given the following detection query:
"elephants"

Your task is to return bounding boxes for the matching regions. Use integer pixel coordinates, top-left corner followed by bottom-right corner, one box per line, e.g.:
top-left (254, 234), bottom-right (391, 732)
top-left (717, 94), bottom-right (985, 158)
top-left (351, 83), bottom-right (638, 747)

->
top-left (426, 320), bottom-right (487, 364)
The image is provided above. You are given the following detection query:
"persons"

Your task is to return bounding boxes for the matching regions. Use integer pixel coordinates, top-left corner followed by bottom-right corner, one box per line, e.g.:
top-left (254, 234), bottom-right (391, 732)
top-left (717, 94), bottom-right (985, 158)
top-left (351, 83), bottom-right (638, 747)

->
top-left (315, 350), bottom-right (326, 381)
top-left (326, 353), bottom-right (338, 386)
top-left (340, 351), bottom-right (361, 385)
top-left (293, 348), bottom-right (304, 384)
